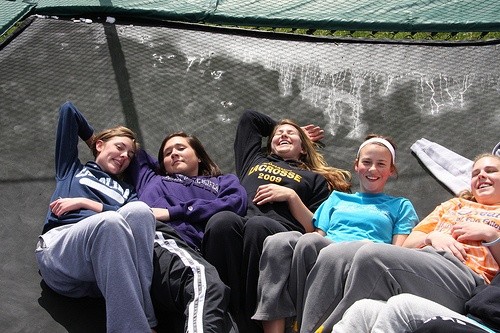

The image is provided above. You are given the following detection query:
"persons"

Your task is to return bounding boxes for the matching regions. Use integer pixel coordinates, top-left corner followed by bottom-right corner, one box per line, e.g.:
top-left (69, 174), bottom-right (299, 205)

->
top-left (251, 135), bottom-right (418, 333)
top-left (35, 101), bottom-right (157, 332)
top-left (203, 111), bottom-right (352, 333)
top-left (332, 273), bottom-right (500, 333)
top-left (128, 133), bottom-right (248, 333)
top-left (300, 154), bottom-right (500, 333)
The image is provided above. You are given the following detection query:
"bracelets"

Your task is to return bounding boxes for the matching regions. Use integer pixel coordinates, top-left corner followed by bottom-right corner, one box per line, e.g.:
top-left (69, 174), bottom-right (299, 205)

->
top-left (481, 237), bottom-right (500, 246)
